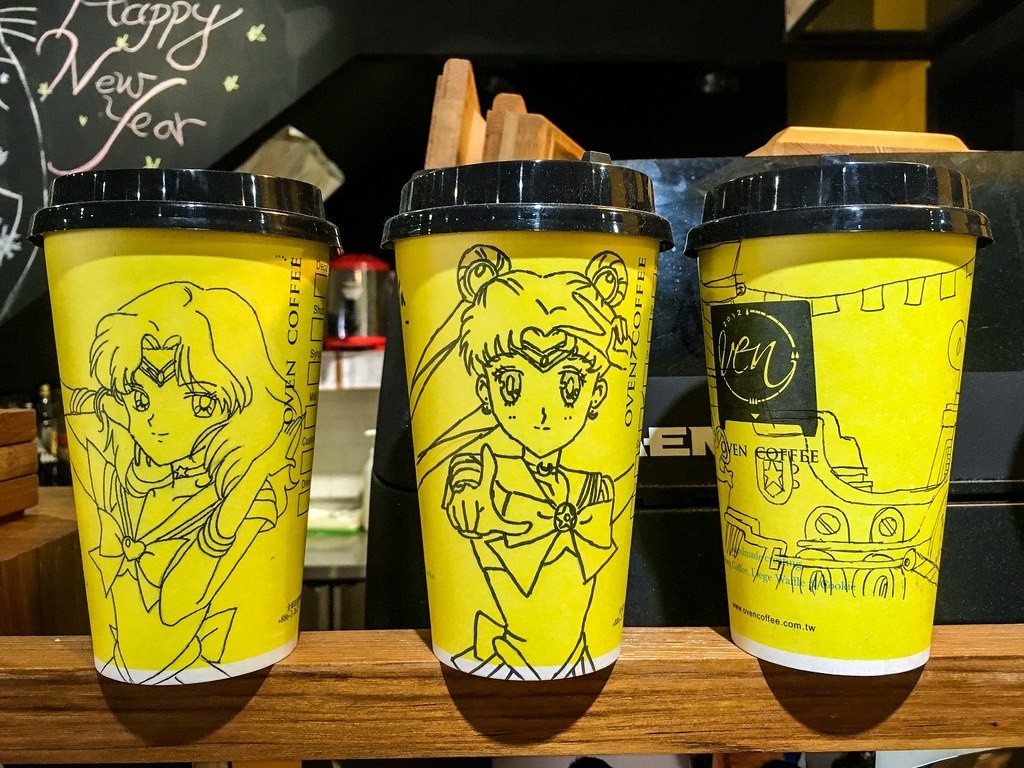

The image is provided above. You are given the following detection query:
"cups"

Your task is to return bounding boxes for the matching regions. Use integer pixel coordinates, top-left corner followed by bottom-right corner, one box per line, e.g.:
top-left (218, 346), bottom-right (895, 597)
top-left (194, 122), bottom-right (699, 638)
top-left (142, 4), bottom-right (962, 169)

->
top-left (24, 167), bottom-right (342, 688)
top-left (377, 160), bottom-right (673, 683)
top-left (683, 160), bottom-right (994, 676)
